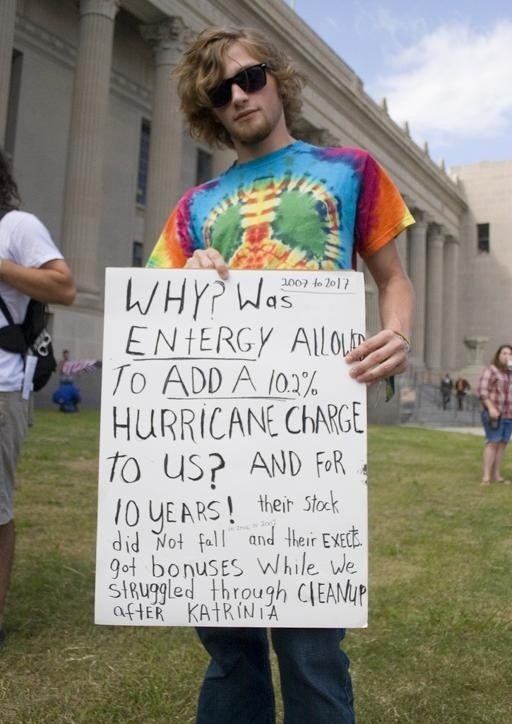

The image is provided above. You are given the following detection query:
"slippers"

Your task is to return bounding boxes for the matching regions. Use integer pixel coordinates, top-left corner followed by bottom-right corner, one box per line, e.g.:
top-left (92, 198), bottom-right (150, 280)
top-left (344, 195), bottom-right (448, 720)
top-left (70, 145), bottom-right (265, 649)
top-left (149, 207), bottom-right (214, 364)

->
top-left (480, 476), bottom-right (510, 486)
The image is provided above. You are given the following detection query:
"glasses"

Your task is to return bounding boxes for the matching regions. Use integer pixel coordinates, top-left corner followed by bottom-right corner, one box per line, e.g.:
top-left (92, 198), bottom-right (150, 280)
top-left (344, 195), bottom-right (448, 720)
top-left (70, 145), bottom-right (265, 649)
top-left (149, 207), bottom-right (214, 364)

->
top-left (201, 63), bottom-right (269, 109)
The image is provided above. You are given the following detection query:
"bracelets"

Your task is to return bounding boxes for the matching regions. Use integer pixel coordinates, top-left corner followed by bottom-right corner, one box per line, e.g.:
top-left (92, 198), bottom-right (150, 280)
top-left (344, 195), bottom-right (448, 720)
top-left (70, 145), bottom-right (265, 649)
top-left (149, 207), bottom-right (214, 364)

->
top-left (394, 332), bottom-right (410, 344)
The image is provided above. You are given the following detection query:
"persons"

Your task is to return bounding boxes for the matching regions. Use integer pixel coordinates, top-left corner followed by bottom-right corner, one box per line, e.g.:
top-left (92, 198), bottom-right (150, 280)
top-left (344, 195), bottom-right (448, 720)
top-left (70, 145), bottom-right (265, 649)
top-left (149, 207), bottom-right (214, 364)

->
top-left (59, 350), bottom-right (73, 385)
top-left (476, 344), bottom-right (512, 486)
top-left (440, 374), bottom-right (453, 410)
top-left (0, 150), bottom-right (76, 641)
top-left (456, 376), bottom-right (471, 410)
top-left (144, 27), bottom-right (417, 724)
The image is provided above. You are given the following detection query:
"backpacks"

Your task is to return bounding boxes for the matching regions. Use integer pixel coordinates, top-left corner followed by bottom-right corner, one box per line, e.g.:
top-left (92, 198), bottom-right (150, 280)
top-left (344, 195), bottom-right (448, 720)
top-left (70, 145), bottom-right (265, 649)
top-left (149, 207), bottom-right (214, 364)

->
top-left (1, 298), bottom-right (56, 393)
